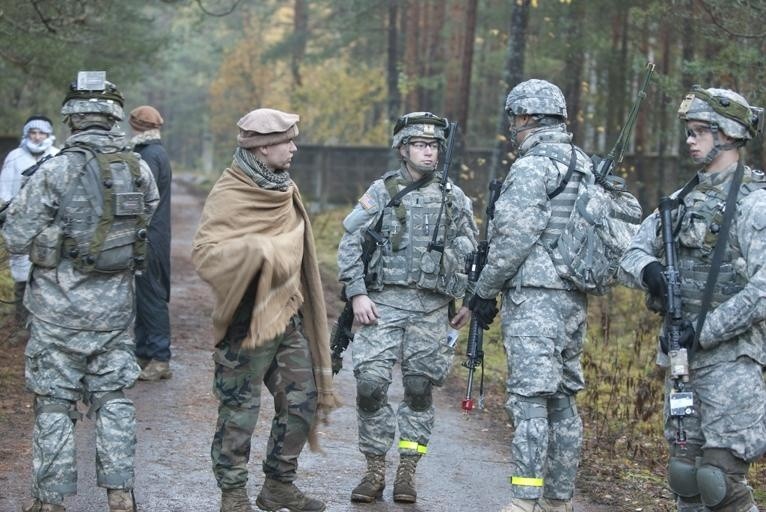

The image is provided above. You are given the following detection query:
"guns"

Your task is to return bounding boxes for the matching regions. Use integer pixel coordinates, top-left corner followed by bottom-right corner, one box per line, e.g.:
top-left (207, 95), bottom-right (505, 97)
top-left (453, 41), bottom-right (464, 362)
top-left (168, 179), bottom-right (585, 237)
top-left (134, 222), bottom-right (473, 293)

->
top-left (658, 195), bottom-right (704, 447)
top-left (330, 215), bottom-right (384, 376)
top-left (462, 178), bottom-right (501, 411)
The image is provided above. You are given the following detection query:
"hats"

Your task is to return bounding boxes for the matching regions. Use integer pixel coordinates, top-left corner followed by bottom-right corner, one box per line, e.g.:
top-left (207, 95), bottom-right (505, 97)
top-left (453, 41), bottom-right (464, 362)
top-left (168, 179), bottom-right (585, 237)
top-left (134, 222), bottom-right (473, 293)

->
top-left (235, 107), bottom-right (300, 149)
top-left (128, 105), bottom-right (163, 133)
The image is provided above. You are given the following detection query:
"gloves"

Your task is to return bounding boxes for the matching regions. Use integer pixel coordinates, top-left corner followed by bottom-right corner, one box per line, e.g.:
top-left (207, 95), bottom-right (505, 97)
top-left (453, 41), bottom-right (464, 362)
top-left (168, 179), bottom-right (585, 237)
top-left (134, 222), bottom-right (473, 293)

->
top-left (643, 262), bottom-right (682, 310)
top-left (469, 293), bottom-right (499, 330)
top-left (659, 321), bottom-right (694, 356)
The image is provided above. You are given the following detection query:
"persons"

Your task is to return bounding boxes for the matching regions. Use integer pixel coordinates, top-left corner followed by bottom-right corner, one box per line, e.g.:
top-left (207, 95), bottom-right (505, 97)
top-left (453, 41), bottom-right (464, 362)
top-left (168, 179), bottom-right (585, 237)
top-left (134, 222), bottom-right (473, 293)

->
top-left (0, 116), bottom-right (60, 325)
top-left (337, 112), bottom-right (479, 502)
top-left (190, 108), bottom-right (341, 511)
top-left (0, 70), bottom-right (160, 511)
top-left (617, 85), bottom-right (766, 512)
top-left (124, 103), bottom-right (173, 384)
top-left (470, 79), bottom-right (593, 511)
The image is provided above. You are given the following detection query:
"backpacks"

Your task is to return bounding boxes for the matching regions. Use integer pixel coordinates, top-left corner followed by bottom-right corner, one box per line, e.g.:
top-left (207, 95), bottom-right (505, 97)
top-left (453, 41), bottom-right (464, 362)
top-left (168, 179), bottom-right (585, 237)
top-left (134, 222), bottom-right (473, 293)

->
top-left (523, 144), bottom-right (643, 297)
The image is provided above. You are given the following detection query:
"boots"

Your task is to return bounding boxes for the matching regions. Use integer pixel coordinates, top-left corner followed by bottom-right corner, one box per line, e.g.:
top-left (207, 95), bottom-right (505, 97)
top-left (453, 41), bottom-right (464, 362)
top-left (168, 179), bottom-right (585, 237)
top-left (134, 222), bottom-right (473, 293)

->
top-left (497, 498), bottom-right (547, 512)
top-left (219, 487), bottom-right (255, 512)
top-left (393, 456), bottom-right (422, 503)
top-left (22, 497), bottom-right (63, 510)
top-left (351, 453), bottom-right (386, 503)
top-left (540, 497), bottom-right (573, 512)
top-left (255, 477), bottom-right (326, 511)
top-left (107, 488), bottom-right (136, 510)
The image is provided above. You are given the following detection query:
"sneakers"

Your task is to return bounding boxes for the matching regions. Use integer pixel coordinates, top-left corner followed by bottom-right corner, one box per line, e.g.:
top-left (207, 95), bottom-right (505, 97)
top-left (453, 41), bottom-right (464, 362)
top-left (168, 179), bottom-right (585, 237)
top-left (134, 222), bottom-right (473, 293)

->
top-left (138, 360), bottom-right (173, 382)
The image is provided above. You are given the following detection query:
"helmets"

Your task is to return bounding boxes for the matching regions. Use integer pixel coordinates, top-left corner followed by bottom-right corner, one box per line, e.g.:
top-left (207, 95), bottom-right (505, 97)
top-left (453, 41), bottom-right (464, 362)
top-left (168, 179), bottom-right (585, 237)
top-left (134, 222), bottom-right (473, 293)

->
top-left (392, 112), bottom-right (447, 155)
top-left (676, 87), bottom-right (753, 141)
top-left (505, 79), bottom-right (568, 120)
top-left (60, 71), bottom-right (127, 123)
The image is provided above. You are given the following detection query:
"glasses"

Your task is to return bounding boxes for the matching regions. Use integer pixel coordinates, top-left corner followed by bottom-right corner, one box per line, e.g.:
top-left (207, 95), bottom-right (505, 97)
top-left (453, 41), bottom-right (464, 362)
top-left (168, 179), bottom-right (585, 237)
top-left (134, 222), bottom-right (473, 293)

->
top-left (684, 124), bottom-right (711, 138)
top-left (408, 139), bottom-right (441, 151)
